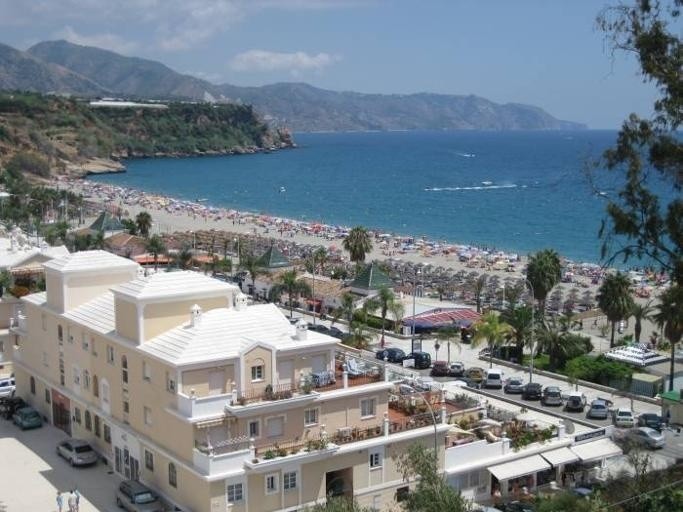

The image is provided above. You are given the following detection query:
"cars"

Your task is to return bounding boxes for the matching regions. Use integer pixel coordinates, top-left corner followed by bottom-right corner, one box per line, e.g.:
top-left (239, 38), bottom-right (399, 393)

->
top-left (375, 348), bottom-right (666, 451)
top-left (55, 438), bottom-right (97, 468)
top-left (0, 378), bottom-right (42, 430)
top-left (116, 480), bottom-right (164, 512)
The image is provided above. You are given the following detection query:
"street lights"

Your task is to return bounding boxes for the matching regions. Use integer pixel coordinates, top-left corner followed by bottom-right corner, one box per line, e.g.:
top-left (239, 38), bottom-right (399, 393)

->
top-left (398, 383), bottom-right (437, 475)
top-left (521, 277), bottom-right (534, 383)
